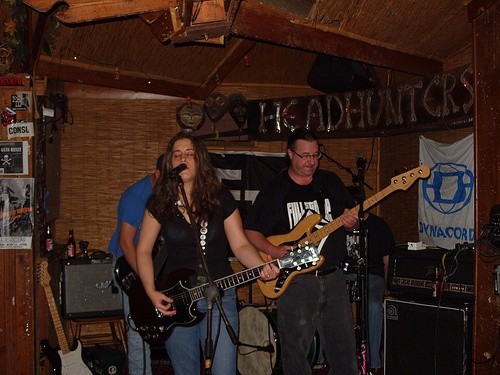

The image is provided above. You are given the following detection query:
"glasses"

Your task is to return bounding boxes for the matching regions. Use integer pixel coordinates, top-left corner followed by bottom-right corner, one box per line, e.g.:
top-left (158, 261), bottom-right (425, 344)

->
top-left (289, 148), bottom-right (321, 159)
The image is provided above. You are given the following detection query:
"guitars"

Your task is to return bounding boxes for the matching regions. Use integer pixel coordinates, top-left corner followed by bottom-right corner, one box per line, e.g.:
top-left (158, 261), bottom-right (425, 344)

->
top-left (255, 159), bottom-right (431, 300)
top-left (36, 260), bottom-right (94, 375)
top-left (127, 240), bottom-right (322, 347)
top-left (114, 242), bottom-right (164, 297)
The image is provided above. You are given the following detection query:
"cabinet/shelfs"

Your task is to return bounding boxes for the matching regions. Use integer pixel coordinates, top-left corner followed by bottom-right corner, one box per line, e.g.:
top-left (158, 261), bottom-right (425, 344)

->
top-left (0, 86), bottom-right (46, 375)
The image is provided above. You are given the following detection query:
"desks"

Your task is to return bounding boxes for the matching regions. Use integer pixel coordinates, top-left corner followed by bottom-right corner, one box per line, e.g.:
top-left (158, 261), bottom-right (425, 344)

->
top-left (72, 315), bottom-right (127, 355)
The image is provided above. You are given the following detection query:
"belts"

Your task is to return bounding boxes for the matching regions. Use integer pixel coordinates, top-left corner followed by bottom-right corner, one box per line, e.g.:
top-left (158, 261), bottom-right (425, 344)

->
top-left (305, 265), bottom-right (341, 276)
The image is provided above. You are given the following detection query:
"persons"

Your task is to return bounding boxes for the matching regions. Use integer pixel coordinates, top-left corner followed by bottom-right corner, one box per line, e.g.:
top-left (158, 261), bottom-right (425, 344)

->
top-left (137, 131), bottom-right (281, 375)
top-left (108, 156), bottom-right (166, 375)
top-left (243, 128), bottom-right (359, 375)
top-left (345, 185), bottom-right (393, 374)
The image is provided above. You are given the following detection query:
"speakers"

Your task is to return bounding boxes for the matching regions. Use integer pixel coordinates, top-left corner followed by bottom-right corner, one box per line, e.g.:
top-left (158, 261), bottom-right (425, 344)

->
top-left (383, 297), bottom-right (474, 375)
top-left (59, 252), bottom-right (125, 320)
top-left (307, 53), bottom-right (381, 94)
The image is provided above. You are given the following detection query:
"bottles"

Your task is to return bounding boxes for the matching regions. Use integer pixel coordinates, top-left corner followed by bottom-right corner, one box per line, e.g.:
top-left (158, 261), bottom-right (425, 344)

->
top-left (66, 230), bottom-right (76, 260)
top-left (45, 224), bottom-right (53, 254)
top-left (433, 263), bottom-right (441, 297)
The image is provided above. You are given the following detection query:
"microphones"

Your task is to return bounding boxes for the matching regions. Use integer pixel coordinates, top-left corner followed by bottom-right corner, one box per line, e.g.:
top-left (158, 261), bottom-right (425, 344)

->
top-left (170, 164), bottom-right (187, 178)
top-left (258, 344), bottom-right (274, 353)
top-left (357, 152), bottom-right (366, 176)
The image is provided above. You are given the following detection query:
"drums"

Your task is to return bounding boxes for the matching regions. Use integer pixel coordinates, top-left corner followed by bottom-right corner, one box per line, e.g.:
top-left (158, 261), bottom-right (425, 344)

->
top-left (235, 306), bottom-right (321, 375)
top-left (345, 279), bottom-right (360, 302)
